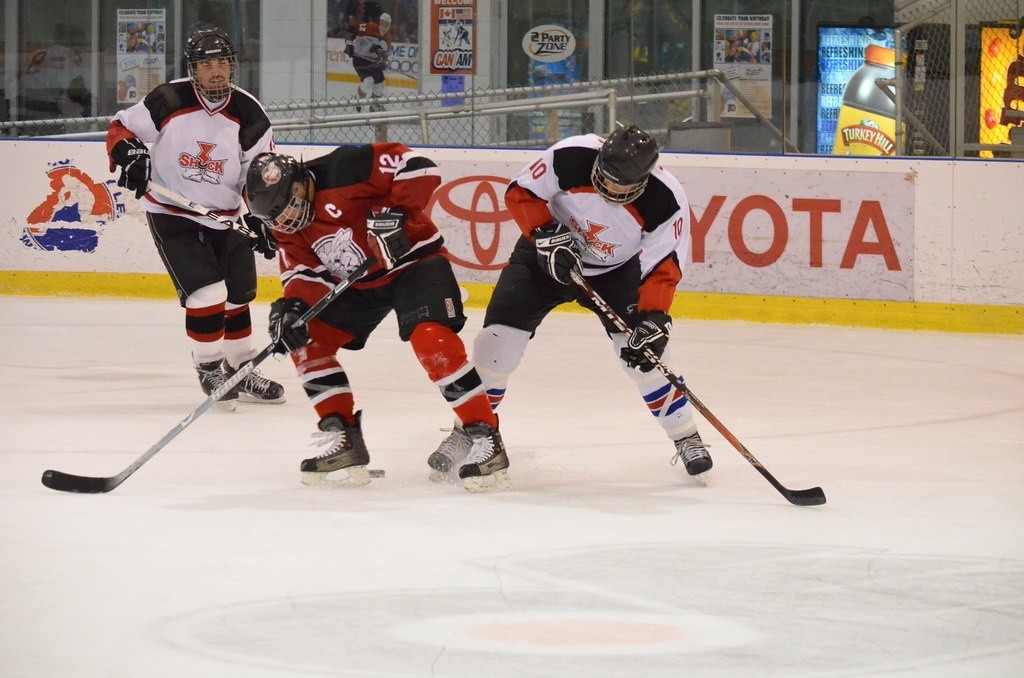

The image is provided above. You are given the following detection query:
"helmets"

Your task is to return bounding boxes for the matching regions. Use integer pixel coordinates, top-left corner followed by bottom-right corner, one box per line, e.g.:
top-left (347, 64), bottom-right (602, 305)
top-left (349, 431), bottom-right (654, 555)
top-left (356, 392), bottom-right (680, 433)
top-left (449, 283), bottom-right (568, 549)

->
top-left (183, 23), bottom-right (239, 103)
top-left (246, 152), bottom-right (317, 234)
top-left (378, 12), bottom-right (391, 37)
top-left (590, 123), bottom-right (659, 206)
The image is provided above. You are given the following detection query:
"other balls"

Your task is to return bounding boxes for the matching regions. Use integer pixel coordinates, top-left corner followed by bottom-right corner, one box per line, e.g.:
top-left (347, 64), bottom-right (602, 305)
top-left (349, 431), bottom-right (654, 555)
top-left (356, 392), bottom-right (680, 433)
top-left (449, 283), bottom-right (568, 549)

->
top-left (368, 469), bottom-right (386, 478)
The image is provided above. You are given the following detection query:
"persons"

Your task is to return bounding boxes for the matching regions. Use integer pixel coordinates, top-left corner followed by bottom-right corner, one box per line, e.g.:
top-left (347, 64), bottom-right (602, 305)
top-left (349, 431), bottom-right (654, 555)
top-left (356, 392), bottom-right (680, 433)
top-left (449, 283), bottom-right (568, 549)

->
top-left (442, 18), bottom-right (470, 48)
top-left (119, 21), bottom-right (165, 53)
top-left (107, 26), bottom-right (288, 413)
top-left (428, 126), bottom-right (715, 487)
top-left (715, 30), bottom-right (770, 63)
top-left (242, 142), bottom-right (510, 491)
top-left (19, 22), bottom-right (92, 136)
top-left (344, 12), bottom-right (392, 113)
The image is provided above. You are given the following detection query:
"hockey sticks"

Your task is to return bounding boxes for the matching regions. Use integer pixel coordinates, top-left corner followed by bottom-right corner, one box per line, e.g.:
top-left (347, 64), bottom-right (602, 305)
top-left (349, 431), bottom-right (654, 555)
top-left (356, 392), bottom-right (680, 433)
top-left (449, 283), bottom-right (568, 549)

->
top-left (567, 265), bottom-right (829, 508)
top-left (145, 179), bottom-right (260, 241)
top-left (39, 250), bottom-right (376, 495)
top-left (376, 60), bottom-right (418, 81)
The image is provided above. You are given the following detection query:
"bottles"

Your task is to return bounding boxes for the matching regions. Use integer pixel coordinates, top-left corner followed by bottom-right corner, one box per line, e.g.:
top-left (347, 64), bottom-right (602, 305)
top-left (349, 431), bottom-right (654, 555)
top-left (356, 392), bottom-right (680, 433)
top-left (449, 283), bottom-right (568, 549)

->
top-left (834, 45), bottom-right (908, 155)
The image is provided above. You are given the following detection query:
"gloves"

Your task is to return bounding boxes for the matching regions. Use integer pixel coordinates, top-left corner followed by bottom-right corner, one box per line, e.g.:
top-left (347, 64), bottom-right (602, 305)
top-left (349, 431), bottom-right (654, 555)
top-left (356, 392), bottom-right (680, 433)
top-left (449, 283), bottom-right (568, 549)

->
top-left (619, 307), bottom-right (672, 373)
top-left (110, 137), bottom-right (153, 199)
top-left (268, 296), bottom-right (309, 354)
top-left (344, 44), bottom-right (354, 57)
top-left (368, 205), bottom-right (411, 270)
top-left (240, 213), bottom-right (278, 259)
top-left (530, 217), bottom-right (584, 286)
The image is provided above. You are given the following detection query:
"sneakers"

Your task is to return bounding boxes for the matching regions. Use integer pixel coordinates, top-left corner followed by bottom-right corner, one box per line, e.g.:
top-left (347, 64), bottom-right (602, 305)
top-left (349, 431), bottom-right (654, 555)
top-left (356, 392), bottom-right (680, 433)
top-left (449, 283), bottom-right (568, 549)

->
top-left (459, 412), bottom-right (512, 493)
top-left (356, 106), bottom-right (361, 113)
top-left (427, 419), bottom-right (474, 483)
top-left (300, 408), bottom-right (371, 492)
top-left (191, 350), bottom-right (240, 413)
top-left (369, 103), bottom-right (386, 112)
top-left (222, 359), bottom-right (287, 404)
top-left (668, 432), bottom-right (713, 488)
